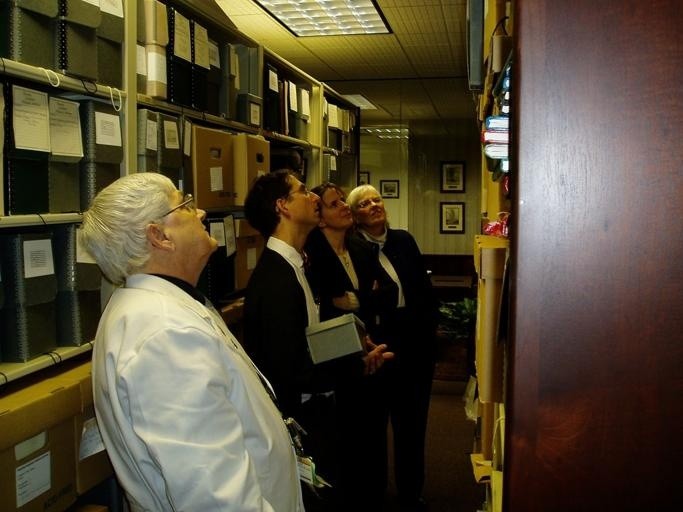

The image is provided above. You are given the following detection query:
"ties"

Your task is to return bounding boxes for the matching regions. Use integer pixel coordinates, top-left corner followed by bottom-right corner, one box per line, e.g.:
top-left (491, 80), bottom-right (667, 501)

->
top-left (300, 261), bottom-right (321, 305)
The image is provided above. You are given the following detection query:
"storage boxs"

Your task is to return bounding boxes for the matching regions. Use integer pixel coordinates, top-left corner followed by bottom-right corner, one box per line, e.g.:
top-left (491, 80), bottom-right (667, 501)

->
top-left (235, 133), bottom-right (271, 205)
top-left (234, 218), bottom-right (267, 289)
top-left (2, 355), bottom-right (112, 509)
top-left (191, 125), bottom-right (235, 208)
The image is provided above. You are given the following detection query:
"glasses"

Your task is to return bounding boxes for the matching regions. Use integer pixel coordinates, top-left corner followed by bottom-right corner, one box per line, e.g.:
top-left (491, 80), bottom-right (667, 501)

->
top-left (158, 193), bottom-right (195, 220)
top-left (356, 196), bottom-right (383, 209)
top-left (289, 185), bottom-right (312, 197)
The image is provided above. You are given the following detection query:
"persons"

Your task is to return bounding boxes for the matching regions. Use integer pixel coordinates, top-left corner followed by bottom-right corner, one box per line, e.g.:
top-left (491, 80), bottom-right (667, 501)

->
top-left (307, 182), bottom-right (385, 511)
top-left (77, 170), bottom-right (306, 510)
top-left (241, 169), bottom-right (395, 511)
top-left (345, 182), bottom-right (441, 510)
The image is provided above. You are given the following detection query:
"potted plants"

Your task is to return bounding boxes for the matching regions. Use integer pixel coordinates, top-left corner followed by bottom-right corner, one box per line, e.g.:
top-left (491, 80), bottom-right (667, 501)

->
top-left (439, 298), bottom-right (476, 365)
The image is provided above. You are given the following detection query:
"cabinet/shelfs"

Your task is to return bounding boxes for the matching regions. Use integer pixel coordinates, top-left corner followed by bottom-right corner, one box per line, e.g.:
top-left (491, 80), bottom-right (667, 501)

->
top-left (0, 2), bottom-right (361, 512)
top-left (473, 0), bottom-right (681, 511)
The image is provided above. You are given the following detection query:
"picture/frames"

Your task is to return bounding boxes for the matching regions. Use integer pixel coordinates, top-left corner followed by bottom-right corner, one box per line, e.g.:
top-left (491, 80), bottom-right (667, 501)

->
top-left (362, 172), bottom-right (398, 199)
top-left (440, 161), bottom-right (465, 194)
top-left (439, 201), bottom-right (465, 234)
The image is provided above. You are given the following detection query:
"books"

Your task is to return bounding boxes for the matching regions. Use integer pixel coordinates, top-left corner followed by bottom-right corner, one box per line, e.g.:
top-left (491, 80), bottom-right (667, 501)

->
top-left (480, 64), bottom-right (510, 181)
